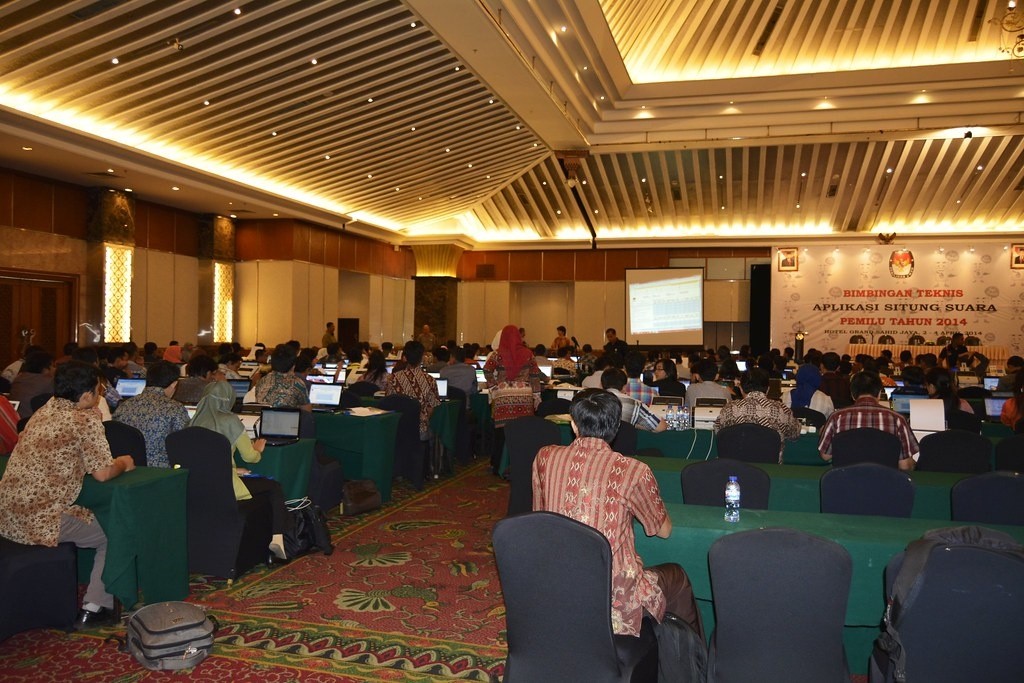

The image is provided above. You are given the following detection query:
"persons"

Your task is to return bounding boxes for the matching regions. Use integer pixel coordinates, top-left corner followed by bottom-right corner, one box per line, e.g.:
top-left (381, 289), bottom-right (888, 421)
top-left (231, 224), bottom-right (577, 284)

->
top-left (1, 322), bottom-right (480, 572)
top-left (484, 325), bottom-right (1024, 479)
top-left (0, 361), bottom-right (136, 627)
top-left (532, 388), bottom-right (708, 664)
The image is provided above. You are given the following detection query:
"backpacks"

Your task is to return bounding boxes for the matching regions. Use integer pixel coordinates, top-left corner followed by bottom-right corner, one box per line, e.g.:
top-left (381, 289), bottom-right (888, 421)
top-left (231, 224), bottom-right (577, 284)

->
top-left (871, 526), bottom-right (1024, 683)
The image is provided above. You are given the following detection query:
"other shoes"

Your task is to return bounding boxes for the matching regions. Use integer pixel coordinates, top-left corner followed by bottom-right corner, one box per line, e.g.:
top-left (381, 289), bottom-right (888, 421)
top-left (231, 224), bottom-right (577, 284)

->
top-left (269, 539), bottom-right (287, 564)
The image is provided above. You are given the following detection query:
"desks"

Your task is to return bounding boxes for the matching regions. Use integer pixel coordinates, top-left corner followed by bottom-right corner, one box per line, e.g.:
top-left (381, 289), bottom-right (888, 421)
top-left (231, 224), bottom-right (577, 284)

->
top-left (627, 499), bottom-right (1024, 675)
top-left (73, 461), bottom-right (190, 611)
top-left (307, 403), bottom-right (398, 507)
top-left (501, 421), bottom-right (826, 480)
top-left (234, 436), bottom-right (316, 506)
top-left (847, 343), bottom-right (1011, 361)
top-left (473, 391), bottom-right (497, 455)
top-left (355, 399), bottom-right (456, 467)
top-left (622, 454), bottom-right (979, 522)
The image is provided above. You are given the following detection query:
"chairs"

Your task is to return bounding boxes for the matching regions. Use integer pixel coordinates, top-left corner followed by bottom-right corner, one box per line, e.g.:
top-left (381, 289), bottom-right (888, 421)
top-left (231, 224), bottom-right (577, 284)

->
top-left (0, 334), bottom-right (1024, 683)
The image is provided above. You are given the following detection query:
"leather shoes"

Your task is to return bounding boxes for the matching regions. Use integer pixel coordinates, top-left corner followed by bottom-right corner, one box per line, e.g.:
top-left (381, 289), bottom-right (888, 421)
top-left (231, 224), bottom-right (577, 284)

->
top-left (78, 606), bottom-right (114, 626)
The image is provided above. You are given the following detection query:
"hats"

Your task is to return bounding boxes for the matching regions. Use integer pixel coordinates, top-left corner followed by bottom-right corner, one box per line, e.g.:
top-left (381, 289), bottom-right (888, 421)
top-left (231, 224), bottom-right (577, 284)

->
top-left (316, 348), bottom-right (328, 360)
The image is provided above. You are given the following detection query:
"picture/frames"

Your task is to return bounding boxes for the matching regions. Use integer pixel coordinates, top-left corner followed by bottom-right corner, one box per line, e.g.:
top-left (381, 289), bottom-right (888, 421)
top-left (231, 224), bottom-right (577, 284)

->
top-left (778, 247), bottom-right (798, 271)
top-left (1010, 243), bottom-right (1024, 269)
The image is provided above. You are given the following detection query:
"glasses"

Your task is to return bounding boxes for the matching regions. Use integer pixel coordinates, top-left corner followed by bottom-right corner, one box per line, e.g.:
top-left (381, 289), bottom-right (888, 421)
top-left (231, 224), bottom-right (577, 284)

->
top-left (656, 368), bottom-right (665, 371)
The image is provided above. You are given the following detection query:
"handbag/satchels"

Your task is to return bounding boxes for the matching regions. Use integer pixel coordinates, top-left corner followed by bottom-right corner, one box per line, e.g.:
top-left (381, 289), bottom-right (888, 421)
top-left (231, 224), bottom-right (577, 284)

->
top-left (126, 601), bottom-right (214, 671)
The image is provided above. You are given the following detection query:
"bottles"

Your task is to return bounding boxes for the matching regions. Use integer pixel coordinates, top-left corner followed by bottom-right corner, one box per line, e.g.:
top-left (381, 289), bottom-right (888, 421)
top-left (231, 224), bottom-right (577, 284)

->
top-left (724, 476), bottom-right (740, 522)
top-left (666, 405), bottom-right (674, 430)
top-left (676, 406), bottom-right (688, 430)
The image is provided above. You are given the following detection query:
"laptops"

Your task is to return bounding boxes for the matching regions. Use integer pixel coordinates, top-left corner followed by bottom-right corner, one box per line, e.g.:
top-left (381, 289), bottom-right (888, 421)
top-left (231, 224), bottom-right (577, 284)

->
top-left (536, 357), bottom-right (797, 432)
top-left (883, 375), bottom-right (1012, 424)
top-left (228, 356), bottom-right (489, 446)
top-left (115, 378), bottom-right (147, 400)
top-left (8, 400), bottom-right (20, 411)
